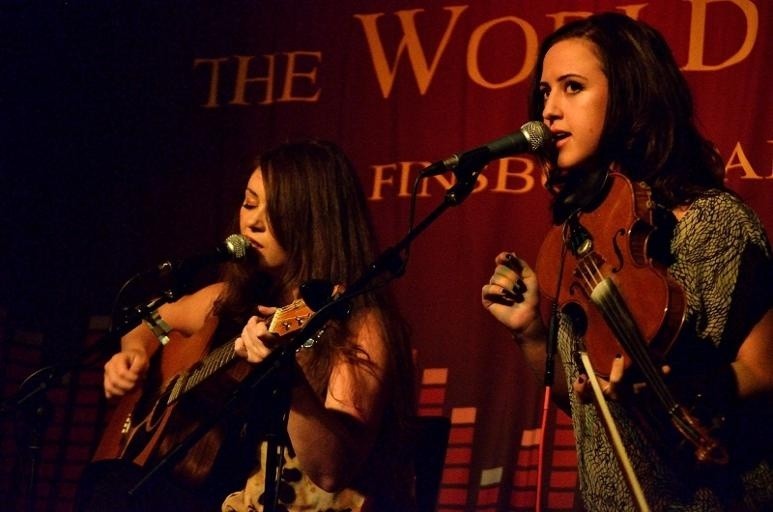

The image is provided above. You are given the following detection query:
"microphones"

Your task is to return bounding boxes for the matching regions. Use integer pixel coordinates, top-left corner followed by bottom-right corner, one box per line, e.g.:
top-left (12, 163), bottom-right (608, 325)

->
top-left (144, 234), bottom-right (251, 274)
top-left (419, 121), bottom-right (551, 179)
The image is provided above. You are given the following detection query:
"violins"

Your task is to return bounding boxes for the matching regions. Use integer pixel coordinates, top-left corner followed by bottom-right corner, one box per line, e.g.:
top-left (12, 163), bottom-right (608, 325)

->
top-left (533, 170), bottom-right (729, 473)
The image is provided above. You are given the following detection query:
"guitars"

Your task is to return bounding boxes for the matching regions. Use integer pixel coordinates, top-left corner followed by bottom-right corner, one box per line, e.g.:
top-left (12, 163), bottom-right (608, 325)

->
top-left (90, 285), bottom-right (345, 511)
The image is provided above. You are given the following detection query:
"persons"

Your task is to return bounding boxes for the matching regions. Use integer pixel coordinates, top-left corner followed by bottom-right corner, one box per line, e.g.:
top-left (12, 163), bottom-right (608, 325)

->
top-left (482, 12), bottom-right (773, 512)
top-left (103, 141), bottom-right (416, 512)
